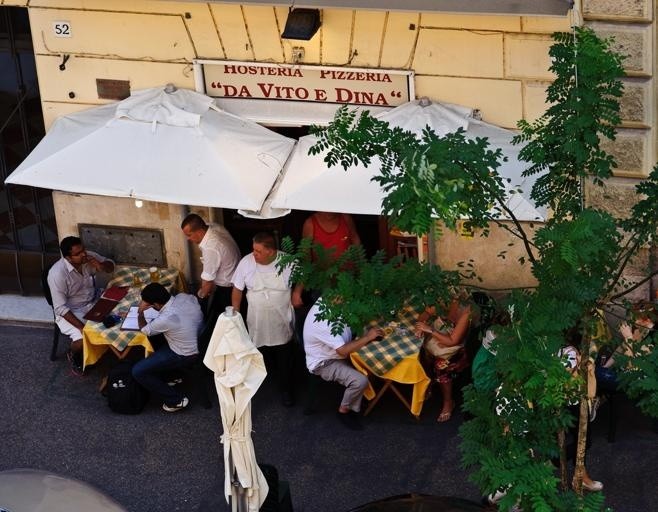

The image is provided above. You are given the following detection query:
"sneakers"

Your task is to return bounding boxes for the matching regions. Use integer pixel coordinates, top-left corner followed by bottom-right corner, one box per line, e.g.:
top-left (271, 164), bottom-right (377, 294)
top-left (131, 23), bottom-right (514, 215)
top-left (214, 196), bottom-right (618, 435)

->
top-left (66, 350), bottom-right (85, 378)
top-left (166, 376), bottom-right (184, 387)
top-left (335, 410), bottom-right (356, 431)
top-left (162, 396), bottom-right (190, 412)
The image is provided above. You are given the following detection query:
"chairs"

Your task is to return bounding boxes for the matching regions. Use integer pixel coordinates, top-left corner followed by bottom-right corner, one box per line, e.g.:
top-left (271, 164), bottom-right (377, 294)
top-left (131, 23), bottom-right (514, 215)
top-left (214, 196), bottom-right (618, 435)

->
top-left (463, 288), bottom-right (496, 354)
top-left (38, 266), bottom-right (74, 361)
top-left (290, 316), bottom-right (323, 414)
top-left (193, 313), bottom-right (217, 408)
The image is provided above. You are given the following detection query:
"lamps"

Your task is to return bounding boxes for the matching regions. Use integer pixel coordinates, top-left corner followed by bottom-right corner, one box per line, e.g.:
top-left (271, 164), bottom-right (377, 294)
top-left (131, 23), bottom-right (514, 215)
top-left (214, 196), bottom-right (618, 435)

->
top-left (280, 7), bottom-right (324, 41)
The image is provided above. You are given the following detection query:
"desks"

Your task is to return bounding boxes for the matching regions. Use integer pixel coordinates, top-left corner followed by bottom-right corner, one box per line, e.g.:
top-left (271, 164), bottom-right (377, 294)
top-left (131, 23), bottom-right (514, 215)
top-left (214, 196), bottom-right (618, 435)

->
top-left (350, 316), bottom-right (426, 420)
top-left (81, 258), bottom-right (188, 391)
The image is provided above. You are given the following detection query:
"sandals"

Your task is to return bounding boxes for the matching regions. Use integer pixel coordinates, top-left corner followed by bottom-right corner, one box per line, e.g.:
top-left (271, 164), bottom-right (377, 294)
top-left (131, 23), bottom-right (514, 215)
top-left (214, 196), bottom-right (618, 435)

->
top-left (437, 399), bottom-right (456, 424)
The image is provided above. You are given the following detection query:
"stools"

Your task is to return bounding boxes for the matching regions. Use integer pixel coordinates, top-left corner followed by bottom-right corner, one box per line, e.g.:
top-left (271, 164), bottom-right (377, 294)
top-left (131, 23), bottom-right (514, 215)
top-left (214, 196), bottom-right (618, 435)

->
top-left (396, 239), bottom-right (419, 264)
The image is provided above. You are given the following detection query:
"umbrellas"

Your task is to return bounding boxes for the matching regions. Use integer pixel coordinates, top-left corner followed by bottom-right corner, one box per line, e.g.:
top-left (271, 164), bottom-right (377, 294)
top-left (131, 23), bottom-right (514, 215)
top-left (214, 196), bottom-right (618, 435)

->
top-left (201, 306), bottom-right (270, 511)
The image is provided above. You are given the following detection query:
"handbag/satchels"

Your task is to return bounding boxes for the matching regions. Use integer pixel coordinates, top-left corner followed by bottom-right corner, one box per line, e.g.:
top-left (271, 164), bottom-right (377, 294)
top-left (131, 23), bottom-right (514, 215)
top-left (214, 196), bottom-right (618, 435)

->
top-left (422, 316), bottom-right (464, 360)
top-left (107, 366), bottom-right (144, 415)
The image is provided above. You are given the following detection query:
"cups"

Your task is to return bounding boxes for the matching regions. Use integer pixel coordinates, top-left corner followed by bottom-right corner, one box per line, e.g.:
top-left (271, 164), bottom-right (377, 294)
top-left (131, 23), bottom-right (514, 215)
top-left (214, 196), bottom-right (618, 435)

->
top-left (149, 267), bottom-right (162, 284)
top-left (133, 273), bottom-right (145, 289)
top-left (382, 326), bottom-right (393, 336)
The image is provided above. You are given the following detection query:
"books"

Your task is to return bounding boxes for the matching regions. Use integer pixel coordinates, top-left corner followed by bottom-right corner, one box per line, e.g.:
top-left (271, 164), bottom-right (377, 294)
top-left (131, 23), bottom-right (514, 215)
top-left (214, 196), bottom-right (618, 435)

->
top-left (121, 305), bottom-right (161, 332)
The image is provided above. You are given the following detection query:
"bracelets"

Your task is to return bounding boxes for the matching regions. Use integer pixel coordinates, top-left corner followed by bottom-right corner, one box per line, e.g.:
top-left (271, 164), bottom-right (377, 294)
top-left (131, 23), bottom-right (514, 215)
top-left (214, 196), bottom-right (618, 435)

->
top-left (429, 327), bottom-right (435, 334)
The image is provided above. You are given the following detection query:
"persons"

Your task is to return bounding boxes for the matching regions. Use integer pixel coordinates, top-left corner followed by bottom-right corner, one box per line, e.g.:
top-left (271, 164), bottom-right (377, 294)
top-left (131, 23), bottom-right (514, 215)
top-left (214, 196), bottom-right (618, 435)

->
top-left (593, 297), bottom-right (657, 384)
top-left (303, 209), bottom-right (365, 293)
top-left (231, 229), bottom-right (307, 407)
top-left (302, 282), bottom-right (386, 431)
top-left (180, 213), bottom-right (242, 339)
top-left (414, 287), bottom-right (477, 425)
top-left (550, 324), bottom-right (604, 493)
top-left (131, 281), bottom-right (206, 412)
top-left (47, 235), bottom-right (117, 376)
